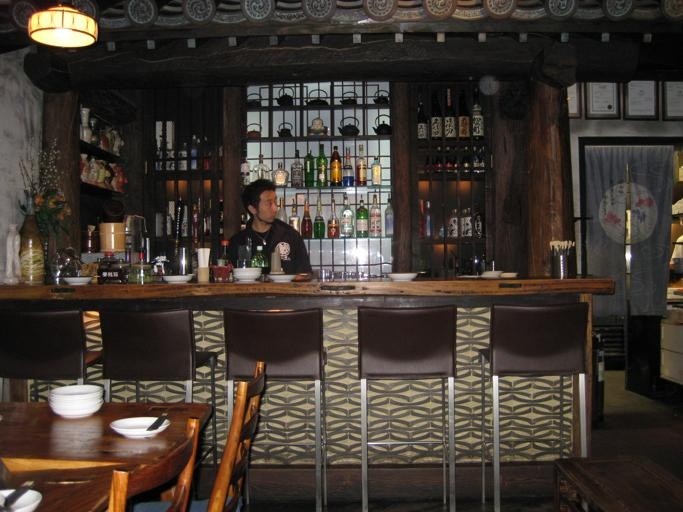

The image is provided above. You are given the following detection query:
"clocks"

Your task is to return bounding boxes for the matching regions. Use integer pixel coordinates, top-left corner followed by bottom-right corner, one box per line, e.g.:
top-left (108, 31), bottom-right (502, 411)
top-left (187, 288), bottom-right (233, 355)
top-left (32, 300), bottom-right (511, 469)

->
top-left (479, 75), bottom-right (500, 97)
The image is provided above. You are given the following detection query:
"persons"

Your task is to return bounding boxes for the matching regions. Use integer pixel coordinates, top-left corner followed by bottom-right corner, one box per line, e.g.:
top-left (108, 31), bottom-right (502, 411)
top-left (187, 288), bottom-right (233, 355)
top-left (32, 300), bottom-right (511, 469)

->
top-left (226, 180), bottom-right (314, 277)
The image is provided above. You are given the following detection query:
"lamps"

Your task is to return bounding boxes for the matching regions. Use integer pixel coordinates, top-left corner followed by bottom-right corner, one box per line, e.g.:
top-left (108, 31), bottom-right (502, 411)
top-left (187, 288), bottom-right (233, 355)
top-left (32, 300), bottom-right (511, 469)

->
top-left (27, 0), bottom-right (98, 49)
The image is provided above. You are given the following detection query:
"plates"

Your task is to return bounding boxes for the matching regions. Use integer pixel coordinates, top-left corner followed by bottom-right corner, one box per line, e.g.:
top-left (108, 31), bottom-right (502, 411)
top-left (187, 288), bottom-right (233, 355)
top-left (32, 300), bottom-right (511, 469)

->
top-left (0, 384), bottom-right (171, 512)
top-left (63, 268), bottom-right (504, 287)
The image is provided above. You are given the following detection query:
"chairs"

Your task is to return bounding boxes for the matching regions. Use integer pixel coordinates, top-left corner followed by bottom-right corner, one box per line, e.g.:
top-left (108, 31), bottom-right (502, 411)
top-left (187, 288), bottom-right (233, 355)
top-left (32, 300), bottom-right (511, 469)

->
top-left (110, 417), bottom-right (201, 512)
top-left (0, 307), bottom-right (102, 401)
top-left (224, 307), bottom-right (327, 512)
top-left (132, 360), bottom-right (266, 512)
top-left (98, 306), bottom-right (216, 501)
top-left (358, 305), bottom-right (456, 512)
top-left (479, 303), bottom-right (589, 512)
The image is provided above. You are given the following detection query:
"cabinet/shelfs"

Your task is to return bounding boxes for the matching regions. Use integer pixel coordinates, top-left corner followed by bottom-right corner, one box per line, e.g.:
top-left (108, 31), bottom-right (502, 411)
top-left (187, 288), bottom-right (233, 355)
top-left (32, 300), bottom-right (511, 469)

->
top-left (660, 319), bottom-right (683, 386)
top-left (39, 83), bottom-right (141, 260)
top-left (625, 299), bottom-right (683, 399)
top-left (223, 77), bottom-right (410, 273)
top-left (142, 80), bottom-right (224, 273)
top-left (408, 79), bottom-right (495, 279)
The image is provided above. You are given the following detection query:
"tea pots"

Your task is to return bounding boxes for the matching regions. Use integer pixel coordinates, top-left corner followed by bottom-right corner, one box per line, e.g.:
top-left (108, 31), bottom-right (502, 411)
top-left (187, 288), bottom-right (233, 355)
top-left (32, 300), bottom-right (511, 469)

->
top-left (246, 87), bottom-right (392, 138)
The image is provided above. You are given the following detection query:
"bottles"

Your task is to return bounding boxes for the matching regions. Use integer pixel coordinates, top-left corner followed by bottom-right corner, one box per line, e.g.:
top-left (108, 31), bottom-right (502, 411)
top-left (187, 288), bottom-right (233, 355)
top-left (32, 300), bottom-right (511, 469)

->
top-left (3, 87), bottom-right (487, 287)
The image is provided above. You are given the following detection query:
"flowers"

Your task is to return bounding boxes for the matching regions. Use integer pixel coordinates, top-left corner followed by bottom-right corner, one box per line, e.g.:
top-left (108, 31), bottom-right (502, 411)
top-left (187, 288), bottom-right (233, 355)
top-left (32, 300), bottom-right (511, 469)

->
top-left (17, 137), bottom-right (74, 240)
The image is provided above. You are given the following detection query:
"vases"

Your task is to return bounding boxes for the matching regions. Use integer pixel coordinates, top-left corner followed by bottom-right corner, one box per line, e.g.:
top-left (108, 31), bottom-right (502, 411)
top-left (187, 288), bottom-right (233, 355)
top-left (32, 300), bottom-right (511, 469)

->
top-left (37, 226), bottom-right (54, 285)
